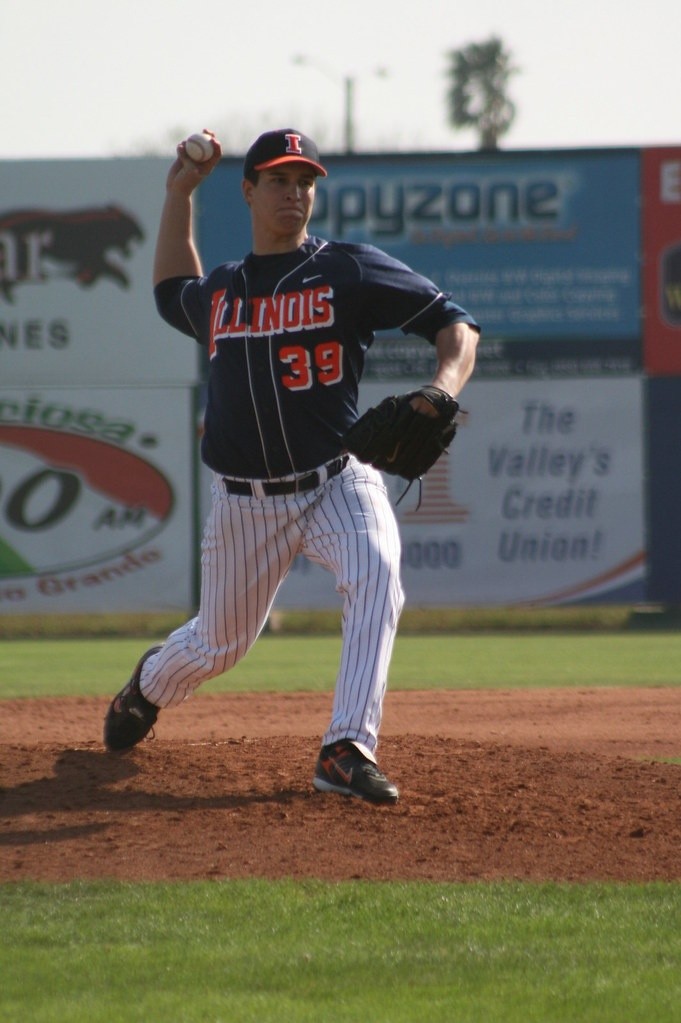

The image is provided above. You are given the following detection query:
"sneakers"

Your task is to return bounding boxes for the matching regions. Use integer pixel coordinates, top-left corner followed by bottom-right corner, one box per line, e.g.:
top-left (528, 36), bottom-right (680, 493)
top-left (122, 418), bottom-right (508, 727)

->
top-left (312, 738), bottom-right (398, 806)
top-left (104, 644), bottom-right (165, 753)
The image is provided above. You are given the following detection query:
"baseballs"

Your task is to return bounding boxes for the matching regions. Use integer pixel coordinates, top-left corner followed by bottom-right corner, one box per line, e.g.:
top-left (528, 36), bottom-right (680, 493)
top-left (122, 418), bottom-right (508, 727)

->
top-left (185, 133), bottom-right (214, 163)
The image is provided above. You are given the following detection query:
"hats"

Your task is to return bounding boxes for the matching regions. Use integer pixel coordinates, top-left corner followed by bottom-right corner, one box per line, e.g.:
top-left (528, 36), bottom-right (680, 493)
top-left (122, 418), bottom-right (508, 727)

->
top-left (243, 129), bottom-right (328, 177)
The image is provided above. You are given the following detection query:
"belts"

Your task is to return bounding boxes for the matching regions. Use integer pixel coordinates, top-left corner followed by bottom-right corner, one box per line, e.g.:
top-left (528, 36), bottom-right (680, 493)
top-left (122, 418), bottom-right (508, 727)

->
top-left (223, 455), bottom-right (348, 496)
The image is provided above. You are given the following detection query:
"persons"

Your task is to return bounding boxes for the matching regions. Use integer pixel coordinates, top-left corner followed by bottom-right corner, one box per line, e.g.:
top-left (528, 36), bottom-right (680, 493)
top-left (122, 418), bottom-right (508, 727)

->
top-left (101, 129), bottom-right (483, 804)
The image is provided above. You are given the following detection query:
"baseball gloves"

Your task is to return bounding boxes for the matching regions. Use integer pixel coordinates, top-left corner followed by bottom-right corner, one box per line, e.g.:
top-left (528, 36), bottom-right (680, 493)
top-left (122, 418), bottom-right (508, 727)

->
top-left (343, 384), bottom-right (459, 481)
